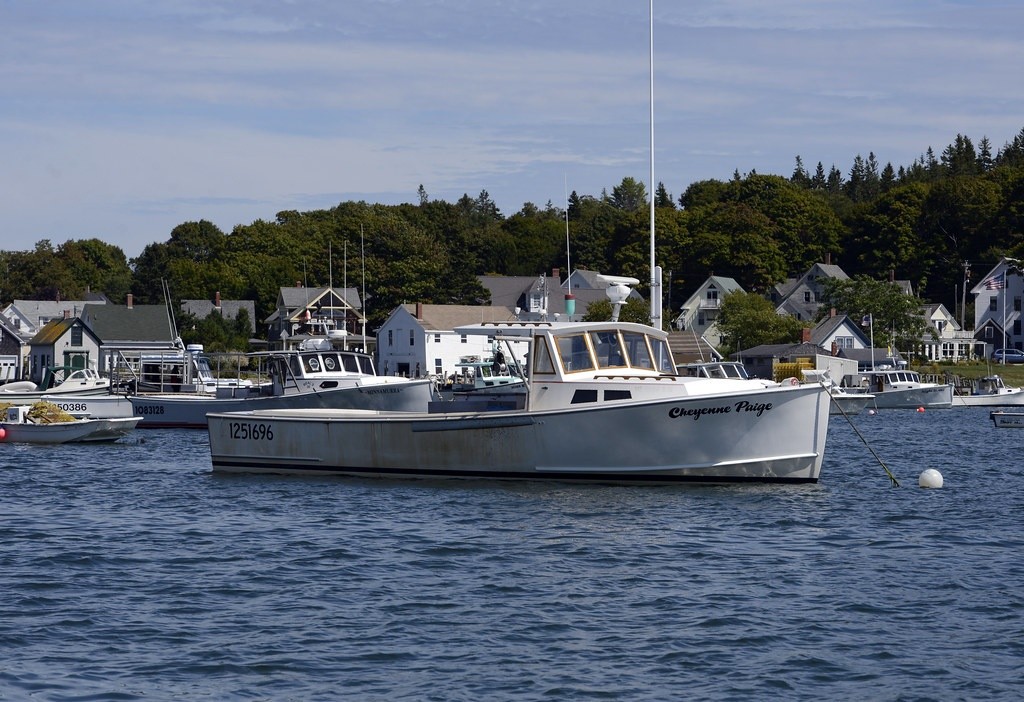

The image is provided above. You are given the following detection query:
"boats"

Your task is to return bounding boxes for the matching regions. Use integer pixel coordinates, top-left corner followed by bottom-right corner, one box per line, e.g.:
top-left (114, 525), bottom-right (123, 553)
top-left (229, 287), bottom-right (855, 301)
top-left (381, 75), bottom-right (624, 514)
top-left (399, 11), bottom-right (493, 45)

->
top-left (202, 0), bottom-right (831, 485)
top-left (129, 345), bottom-right (253, 398)
top-left (677, 360), bottom-right (876, 416)
top-left (864, 361), bottom-right (956, 409)
top-left (0, 368), bottom-right (136, 419)
top-left (114, 342), bottom-right (433, 425)
top-left (919, 364), bottom-right (1024, 407)
top-left (988, 409), bottom-right (1024, 428)
top-left (449, 360), bottom-right (529, 398)
top-left (0, 407), bottom-right (144, 445)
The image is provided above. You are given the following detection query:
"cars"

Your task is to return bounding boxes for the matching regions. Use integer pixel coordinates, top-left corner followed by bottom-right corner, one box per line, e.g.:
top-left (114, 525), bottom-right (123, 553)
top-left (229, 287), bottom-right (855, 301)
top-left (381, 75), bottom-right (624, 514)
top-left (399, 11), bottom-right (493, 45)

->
top-left (991, 348), bottom-right (1024, 365)
